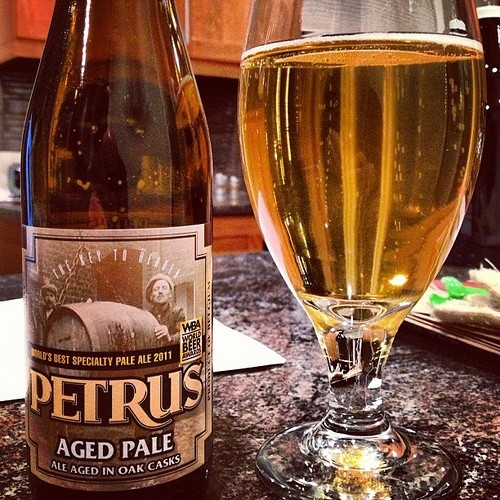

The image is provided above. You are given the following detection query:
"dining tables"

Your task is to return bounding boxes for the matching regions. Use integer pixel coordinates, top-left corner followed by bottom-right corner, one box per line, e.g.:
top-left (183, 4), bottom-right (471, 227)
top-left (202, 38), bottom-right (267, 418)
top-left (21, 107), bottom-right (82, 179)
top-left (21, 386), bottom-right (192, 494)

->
top-left (0, 251), bottom-right (500, 500)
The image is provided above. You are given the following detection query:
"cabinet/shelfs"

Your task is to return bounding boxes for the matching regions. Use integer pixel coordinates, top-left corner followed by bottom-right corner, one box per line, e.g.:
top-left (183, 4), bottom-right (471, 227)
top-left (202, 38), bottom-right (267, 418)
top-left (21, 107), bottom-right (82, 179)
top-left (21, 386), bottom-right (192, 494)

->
top-left (1, 0), bottom-right (303, 253)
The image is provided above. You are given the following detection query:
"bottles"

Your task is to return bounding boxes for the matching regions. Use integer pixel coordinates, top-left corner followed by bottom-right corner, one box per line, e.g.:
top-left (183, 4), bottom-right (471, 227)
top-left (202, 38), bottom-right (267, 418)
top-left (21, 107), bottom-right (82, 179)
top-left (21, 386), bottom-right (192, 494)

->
top-left (19, 0), bottom-right (215, 498)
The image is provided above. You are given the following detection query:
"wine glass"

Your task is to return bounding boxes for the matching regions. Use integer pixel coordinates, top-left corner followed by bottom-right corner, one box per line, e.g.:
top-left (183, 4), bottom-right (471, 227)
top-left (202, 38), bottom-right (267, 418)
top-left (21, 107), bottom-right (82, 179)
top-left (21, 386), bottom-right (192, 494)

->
top-left (238, 0), bottom-right (486, 500)
